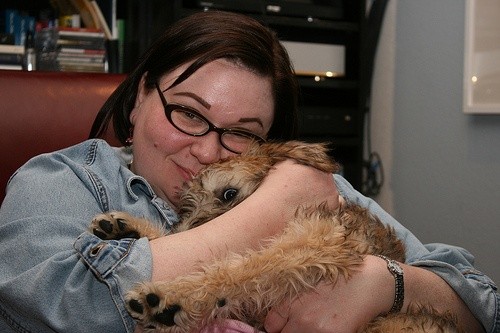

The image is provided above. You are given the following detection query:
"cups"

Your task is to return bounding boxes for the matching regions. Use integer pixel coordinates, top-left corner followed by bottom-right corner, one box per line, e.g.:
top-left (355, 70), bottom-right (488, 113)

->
top-left (35, 28), bottom-right (56, 73)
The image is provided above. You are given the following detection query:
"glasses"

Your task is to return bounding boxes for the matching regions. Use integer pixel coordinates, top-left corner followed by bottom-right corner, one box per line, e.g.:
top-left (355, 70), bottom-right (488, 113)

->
top-left (155, 84), bottom-right (267, 154)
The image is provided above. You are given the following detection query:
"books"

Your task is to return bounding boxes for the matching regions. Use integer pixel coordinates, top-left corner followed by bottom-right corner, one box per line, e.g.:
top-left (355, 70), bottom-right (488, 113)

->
top-left (56, 27), bottom-right (106, 74)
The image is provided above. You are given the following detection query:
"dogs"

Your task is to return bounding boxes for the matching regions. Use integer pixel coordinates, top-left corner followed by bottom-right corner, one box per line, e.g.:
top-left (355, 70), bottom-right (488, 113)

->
top-left (88, 138), bottom-right (458, 333)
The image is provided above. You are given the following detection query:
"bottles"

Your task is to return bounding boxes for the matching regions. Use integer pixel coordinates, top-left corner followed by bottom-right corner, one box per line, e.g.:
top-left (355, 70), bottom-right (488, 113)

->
top-left (23, 19), bottom-right (48, 72)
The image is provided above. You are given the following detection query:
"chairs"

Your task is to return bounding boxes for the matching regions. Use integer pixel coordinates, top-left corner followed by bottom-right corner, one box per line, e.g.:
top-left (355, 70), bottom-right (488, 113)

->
top-left (0, 70), bottom-right (132, 209)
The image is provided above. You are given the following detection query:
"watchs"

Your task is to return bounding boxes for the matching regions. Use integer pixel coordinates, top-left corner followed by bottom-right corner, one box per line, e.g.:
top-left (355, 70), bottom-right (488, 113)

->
top-left (376, 255), bottom-right (404, 313)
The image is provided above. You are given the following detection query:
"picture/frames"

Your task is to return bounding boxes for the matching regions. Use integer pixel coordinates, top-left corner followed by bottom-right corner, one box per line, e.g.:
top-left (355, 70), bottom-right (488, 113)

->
top-left (462, 0), bottom-right (500, 116)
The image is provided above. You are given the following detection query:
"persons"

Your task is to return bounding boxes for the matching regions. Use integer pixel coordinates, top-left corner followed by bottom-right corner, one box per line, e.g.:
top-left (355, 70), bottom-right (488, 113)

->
top-left (0, 11), bottom-right (499, 332)
